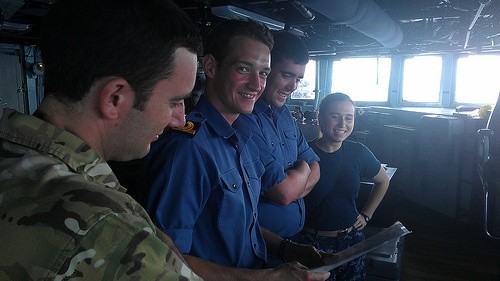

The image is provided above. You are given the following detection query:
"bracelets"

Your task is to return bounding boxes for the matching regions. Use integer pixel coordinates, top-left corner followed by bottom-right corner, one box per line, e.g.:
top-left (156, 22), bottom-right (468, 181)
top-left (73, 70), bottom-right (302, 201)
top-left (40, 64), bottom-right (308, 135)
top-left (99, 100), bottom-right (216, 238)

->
top-left (359, 213), bottom-right (370, 223)
top-left (277, 239), bottom-right (292, 263)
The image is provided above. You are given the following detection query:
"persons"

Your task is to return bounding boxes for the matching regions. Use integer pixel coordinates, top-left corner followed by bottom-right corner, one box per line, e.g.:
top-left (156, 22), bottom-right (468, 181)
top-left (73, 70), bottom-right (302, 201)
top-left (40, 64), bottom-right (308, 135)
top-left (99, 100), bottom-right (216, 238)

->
top-left (125, 17), bottom-right (338, 280)
top-left (1, 0), bottom-right (205, 280)
top-left (238, 29), bottom-right (321, 268)
top-left (289, 93), bottom-right (390, 281)
